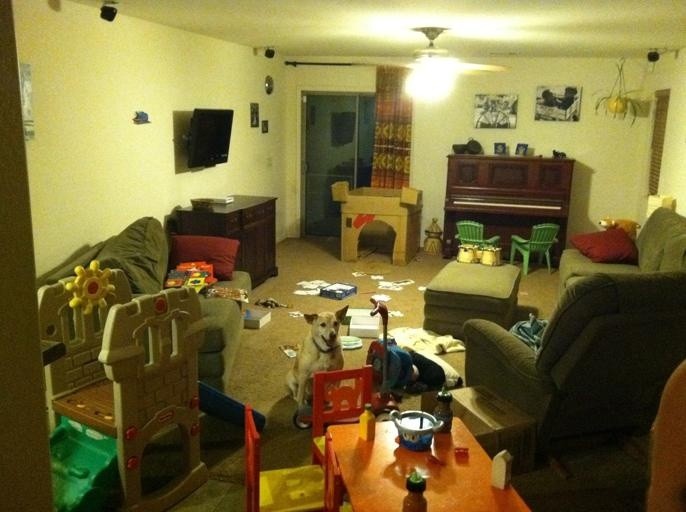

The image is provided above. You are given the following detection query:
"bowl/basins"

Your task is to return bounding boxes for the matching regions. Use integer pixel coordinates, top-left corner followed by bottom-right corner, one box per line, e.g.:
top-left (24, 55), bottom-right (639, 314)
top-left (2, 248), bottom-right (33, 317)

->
top-left (338, 335), bottom-right (362, 348)
top-left (457, 244), bottom-right (504, 268)
top-left (454, 144), bottom-right (466, 154)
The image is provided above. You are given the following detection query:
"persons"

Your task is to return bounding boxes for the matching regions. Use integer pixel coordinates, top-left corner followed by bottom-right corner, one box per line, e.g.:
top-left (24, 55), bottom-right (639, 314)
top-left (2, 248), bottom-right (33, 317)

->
top-left (542, 85), bottom-right (577, 110)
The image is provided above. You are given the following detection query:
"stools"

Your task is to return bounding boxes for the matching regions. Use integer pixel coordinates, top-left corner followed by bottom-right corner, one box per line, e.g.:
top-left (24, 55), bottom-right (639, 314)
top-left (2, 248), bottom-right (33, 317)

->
top-left (421, 255), bottom-right (522, 345)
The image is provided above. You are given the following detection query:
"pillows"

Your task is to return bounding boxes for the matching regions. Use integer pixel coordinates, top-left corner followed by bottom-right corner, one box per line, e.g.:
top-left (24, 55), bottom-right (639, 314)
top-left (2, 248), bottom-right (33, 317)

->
top-left (168, 234), bottom-right (242, 281)
top-left (571, 226), bottom-right (641, 265)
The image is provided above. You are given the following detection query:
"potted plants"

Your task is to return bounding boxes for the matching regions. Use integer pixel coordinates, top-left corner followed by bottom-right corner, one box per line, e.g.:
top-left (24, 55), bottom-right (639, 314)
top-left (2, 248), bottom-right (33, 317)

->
top-left (595, 55), bottom-right (645, 125)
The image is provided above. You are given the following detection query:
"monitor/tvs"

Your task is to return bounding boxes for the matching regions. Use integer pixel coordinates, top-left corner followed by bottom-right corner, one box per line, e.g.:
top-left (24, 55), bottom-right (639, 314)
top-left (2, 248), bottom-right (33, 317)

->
top-left (187, 108), bottom-right (234, 168)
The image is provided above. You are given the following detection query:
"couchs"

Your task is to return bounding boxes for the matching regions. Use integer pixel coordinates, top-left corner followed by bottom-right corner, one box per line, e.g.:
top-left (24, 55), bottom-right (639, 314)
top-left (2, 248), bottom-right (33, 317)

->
top-left (555, 203), bottom-right (686, 297)
top-left (46, 210), bottom-right (256, 401)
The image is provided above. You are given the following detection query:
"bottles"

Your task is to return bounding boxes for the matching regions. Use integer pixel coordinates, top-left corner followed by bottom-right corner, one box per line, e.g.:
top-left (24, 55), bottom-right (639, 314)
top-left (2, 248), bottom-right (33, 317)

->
top-left (433, 385), bottom-right (454, 436)
top-left (358, 402), bottom-right (377, 443)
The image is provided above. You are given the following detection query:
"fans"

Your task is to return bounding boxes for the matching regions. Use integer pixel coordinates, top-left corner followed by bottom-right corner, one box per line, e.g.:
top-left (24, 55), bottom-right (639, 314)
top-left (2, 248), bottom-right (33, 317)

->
top-left (393, 24), bottom-right (507, 73)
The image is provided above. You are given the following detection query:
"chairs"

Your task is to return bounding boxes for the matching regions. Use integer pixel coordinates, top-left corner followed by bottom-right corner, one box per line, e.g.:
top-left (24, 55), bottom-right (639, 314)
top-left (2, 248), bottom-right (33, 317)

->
top-left (239, 401), bottom-right (338, 512)
top-left (457, 264), bottom-right (685, 458)
top-left (506, 222), bottom-right (563, 279)
top-left (454, 219), bottom-right (500, 249)
top-left (307, 365), bottom-right (380, 471)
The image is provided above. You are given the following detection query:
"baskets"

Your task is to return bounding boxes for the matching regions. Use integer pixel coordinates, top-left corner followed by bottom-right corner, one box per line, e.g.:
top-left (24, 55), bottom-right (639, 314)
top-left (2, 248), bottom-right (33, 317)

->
top-left (456, 242), bottom-right (502, 266)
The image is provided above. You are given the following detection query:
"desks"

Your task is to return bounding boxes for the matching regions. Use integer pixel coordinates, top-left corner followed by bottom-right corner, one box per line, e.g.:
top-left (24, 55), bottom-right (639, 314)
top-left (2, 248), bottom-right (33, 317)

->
top-left (321, 413), bottom-right (532, 512)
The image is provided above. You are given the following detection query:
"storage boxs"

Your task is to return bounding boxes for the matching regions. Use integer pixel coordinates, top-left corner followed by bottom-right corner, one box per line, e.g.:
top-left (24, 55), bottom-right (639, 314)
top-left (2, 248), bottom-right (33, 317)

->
top-left (445, 384), bottom-right (538, 476)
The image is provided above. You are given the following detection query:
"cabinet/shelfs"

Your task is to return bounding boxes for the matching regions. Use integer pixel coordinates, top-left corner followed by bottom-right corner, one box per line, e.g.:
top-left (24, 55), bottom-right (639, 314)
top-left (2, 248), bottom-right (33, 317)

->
top-left (175, 192), bottom-right (279, 290)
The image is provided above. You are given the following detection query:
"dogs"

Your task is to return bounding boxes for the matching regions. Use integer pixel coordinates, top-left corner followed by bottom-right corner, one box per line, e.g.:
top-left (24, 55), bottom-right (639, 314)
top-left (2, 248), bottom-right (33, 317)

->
top-left (286, 305), bottom-right (350, 411)
